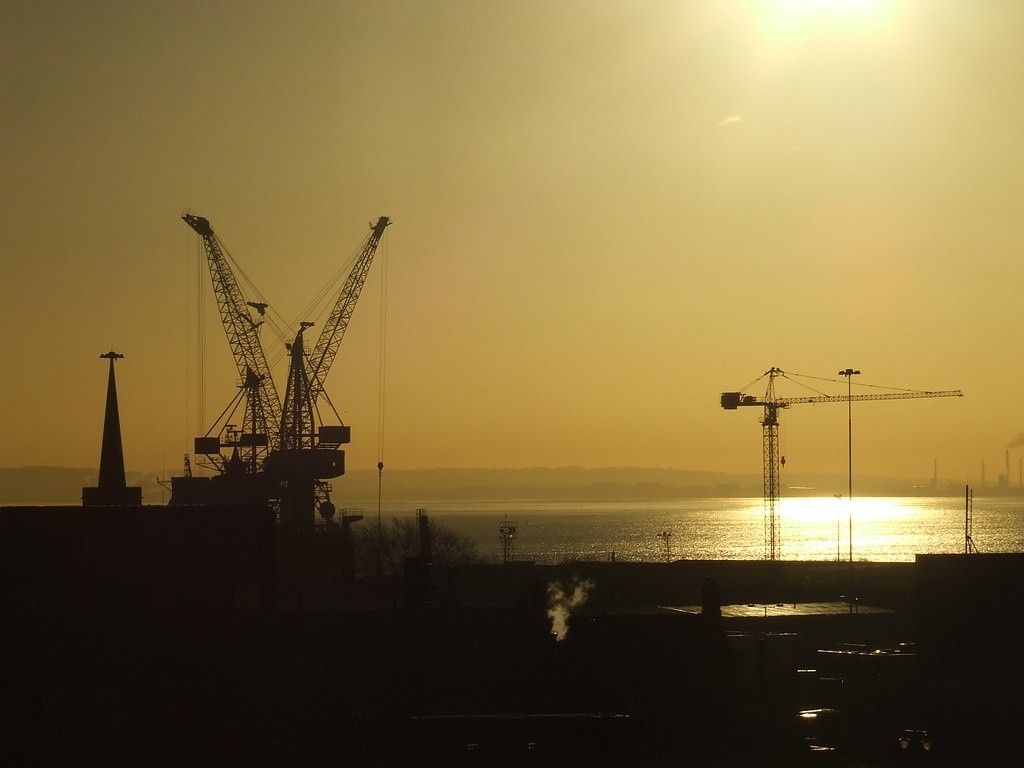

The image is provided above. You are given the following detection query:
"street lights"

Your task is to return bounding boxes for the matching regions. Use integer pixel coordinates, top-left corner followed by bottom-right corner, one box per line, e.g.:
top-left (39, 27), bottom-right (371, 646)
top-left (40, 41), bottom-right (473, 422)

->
top-left (837, 369), bottom-right (861, 594)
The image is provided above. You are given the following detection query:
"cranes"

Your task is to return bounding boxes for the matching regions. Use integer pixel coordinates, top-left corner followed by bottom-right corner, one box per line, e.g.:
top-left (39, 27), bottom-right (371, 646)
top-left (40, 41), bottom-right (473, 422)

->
top-left (179, 206), bottom-right (394, 570)
top-left (718, 365), bottom-right (965, 562)
